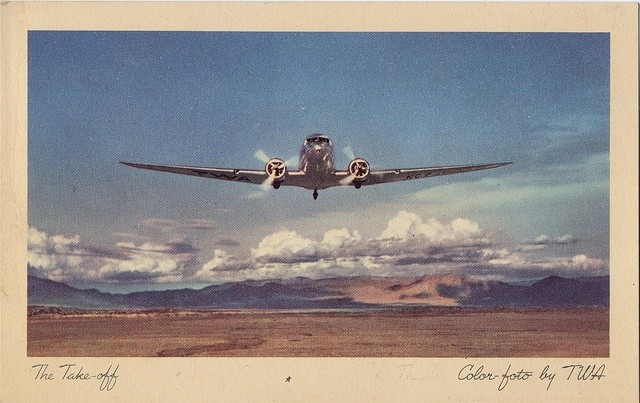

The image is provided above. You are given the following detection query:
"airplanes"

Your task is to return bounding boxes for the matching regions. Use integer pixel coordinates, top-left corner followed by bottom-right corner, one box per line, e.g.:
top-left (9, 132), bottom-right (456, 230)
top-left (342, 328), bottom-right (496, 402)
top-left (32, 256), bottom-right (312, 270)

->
top-left (119, 134), bottom-right (513, 197)
